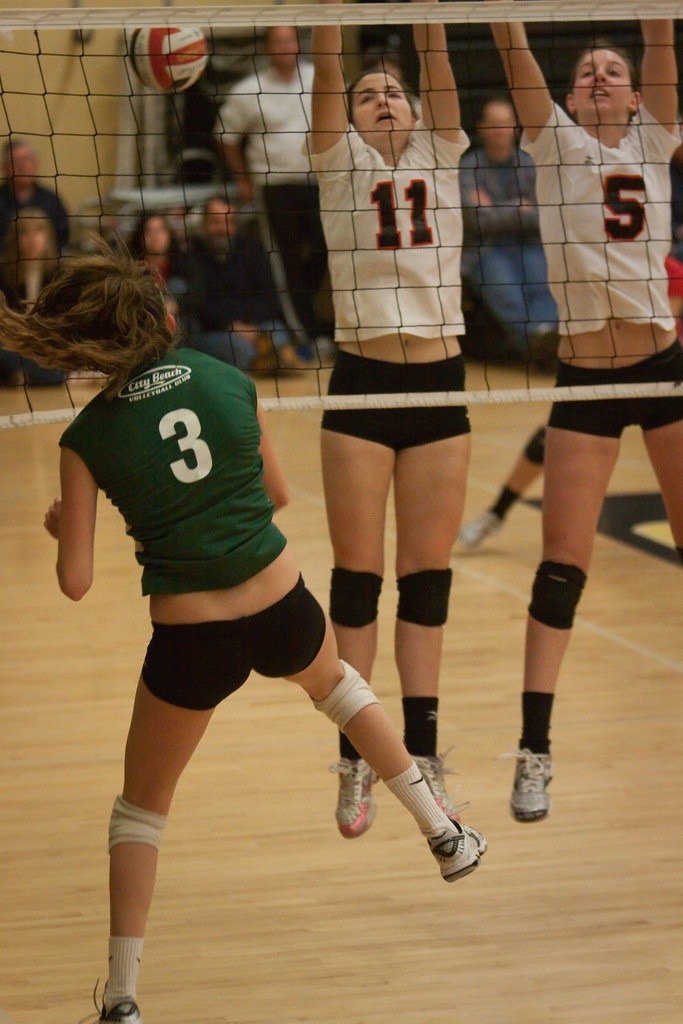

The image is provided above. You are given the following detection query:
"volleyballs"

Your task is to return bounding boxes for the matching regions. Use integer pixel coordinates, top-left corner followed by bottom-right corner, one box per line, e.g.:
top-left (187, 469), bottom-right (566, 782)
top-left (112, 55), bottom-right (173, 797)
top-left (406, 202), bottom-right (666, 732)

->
top-left (126, 24), bottom-right (209, 90)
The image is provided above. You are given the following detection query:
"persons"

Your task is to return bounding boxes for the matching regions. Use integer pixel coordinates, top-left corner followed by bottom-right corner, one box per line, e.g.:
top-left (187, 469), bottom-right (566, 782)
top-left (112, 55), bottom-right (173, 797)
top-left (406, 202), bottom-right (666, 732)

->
top-left (0, 24), bottom-right (338, 385)
top-left (458, 99), bottom-right (560, 367)
top-left (0, 254), bottom-right (486, 1023)
top-left (460, 256), bottom-right (683, 545)
top-left (489, 19), bottom-right (683, 822)
top-left (311, 21), bottom-right (469, 840)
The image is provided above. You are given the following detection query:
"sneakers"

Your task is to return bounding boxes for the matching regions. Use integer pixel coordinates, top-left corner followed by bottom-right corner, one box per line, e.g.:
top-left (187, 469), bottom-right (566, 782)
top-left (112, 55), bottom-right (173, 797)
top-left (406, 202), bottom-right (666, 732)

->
top-left (425, 818), bottom-right (485, 883)
top-left (410, 756), bottom-right (469, 826)
top-left (93, 977), bottom-right (140, 1024)
top-left (501, 749), bottom-right (553, 823)
top-left (327, 758), bottom-right (379, 839)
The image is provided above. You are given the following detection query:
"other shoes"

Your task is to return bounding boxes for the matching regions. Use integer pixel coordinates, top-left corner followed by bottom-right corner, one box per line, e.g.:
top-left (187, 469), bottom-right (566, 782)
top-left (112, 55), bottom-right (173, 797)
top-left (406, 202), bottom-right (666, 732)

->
top-left (459, 511), bottom-right (504, 549)
